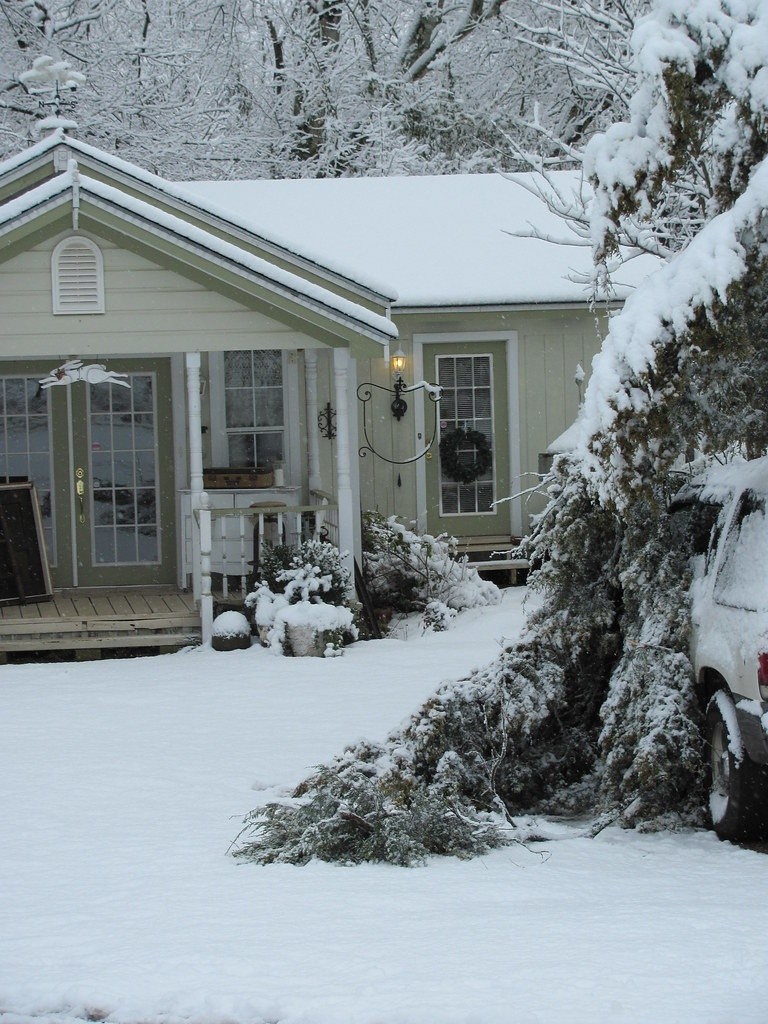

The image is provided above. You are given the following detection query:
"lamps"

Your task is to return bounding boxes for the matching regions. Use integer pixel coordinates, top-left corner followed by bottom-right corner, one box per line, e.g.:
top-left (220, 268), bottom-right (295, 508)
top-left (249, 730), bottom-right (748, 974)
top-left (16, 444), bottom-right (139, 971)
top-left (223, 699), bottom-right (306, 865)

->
top-left (390, 342), bottom-right (408, 421)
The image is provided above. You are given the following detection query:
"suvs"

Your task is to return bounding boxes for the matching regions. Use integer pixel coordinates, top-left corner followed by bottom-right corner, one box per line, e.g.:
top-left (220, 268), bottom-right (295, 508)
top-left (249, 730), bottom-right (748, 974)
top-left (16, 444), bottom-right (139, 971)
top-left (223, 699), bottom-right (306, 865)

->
top-left (663, 457), bottom-right (768, 844)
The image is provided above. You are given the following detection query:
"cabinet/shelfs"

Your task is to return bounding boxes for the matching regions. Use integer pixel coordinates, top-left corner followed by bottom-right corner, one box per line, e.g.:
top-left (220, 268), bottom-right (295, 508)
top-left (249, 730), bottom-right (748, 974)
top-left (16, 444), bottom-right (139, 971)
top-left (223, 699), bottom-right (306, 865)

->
top-left (179, 486), bottom-right (300, 600)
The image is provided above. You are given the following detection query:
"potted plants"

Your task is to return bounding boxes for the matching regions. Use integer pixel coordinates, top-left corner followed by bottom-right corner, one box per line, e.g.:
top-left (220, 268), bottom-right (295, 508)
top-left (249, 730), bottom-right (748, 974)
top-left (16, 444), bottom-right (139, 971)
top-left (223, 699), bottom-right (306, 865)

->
top-left (440, 428), bottom-right (493, 483)
